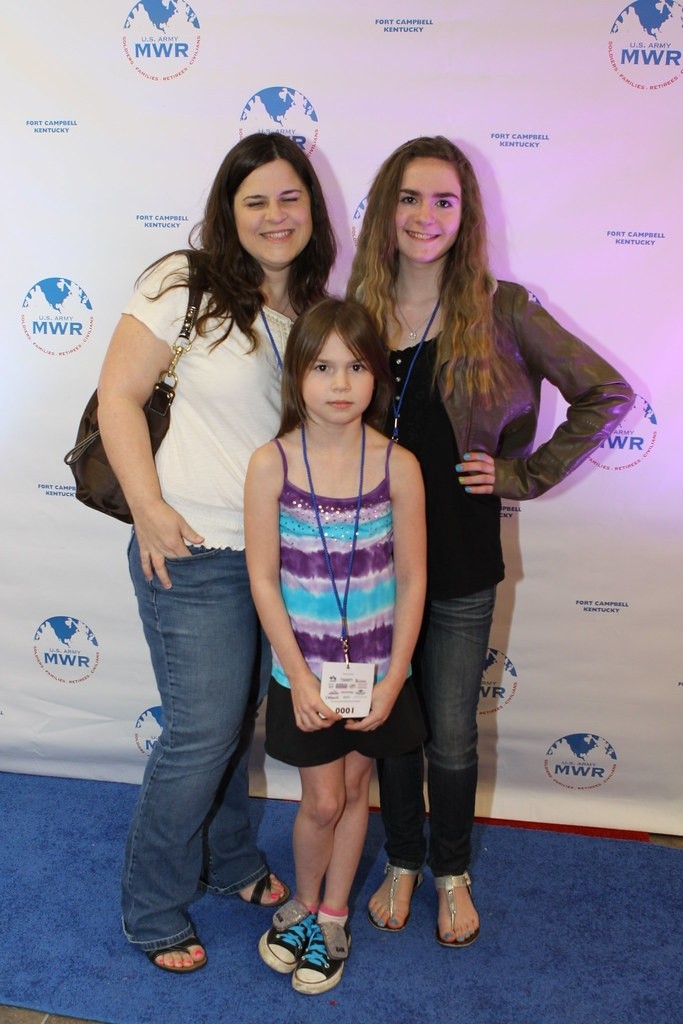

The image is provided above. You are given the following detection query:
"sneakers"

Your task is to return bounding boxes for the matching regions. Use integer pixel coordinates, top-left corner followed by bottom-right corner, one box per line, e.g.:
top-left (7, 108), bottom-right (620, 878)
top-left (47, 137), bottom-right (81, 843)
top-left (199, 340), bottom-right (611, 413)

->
top-left (258, 899), bottom-right (318, 974)
top-left (292, 920), bottom-right (351, 994)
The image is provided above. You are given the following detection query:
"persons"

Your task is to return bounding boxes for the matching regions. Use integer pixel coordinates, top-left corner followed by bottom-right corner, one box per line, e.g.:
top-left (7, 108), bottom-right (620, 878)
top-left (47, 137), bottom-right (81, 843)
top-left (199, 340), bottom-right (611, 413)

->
top-left (243, 300), bottom-right (424, 995)
top-left (98, 133), bottom-right (336, 974)
top-left (344, 135), bottom-right (635, 945)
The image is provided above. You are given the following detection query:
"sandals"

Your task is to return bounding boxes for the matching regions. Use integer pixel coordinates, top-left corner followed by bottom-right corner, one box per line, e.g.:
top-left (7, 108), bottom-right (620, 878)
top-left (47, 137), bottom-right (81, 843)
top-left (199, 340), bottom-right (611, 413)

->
top-left (234, 872), bottom-right (290, 906)
top-left (367, 859), bottom-right (423, 931)
top-left (145, 936), bottom-right (208, 972)
top-left (433, 874), bottom-right (480, 947)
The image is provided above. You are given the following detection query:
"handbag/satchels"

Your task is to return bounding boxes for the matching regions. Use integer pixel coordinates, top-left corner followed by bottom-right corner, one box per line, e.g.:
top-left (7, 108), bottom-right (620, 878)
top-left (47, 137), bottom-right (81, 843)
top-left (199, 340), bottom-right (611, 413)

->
top-left (64, 252), bottom-right (202, 526)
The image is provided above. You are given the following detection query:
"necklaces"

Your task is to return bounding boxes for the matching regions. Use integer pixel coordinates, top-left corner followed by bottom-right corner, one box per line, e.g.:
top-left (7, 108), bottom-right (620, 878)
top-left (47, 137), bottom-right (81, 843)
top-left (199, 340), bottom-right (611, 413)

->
top-left (281, 302), bottom-right (290, 314)
top-left (393, 280), bottom-right (432, 340)
top-left (259, 306), bottom-right (283, 372)
top-left (391, 296), bottom-right (441, 444)
